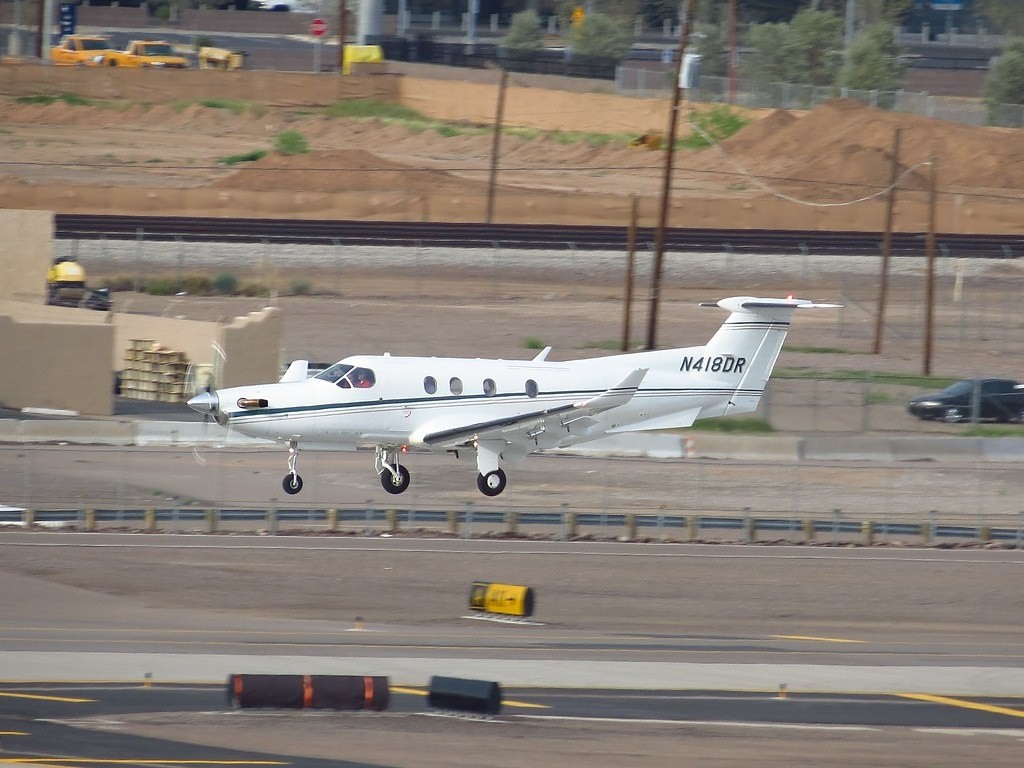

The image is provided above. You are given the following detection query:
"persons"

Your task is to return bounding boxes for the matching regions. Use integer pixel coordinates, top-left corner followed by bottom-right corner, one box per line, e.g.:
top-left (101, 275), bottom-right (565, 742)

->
top-left (343, 370), bottom-right (372, 388)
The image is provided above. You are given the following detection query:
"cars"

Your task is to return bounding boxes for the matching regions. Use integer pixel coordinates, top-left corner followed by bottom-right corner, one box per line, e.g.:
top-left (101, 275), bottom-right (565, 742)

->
top-left (50, 35), bottom-right (142, 68)
top-left (115, 38), bottom-right (192, 69)
top-left (906, 376), bottom-right (1024, 425)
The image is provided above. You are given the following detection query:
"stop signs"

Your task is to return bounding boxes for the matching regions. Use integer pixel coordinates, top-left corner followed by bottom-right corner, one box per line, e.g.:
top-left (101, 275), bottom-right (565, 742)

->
top-left (309, 19), bottom-right (327, 37)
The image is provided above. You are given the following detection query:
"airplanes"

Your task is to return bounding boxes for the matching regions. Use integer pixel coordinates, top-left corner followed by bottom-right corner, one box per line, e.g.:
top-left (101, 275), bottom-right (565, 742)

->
top-left (184, 287), bottom-right (846, 496)
